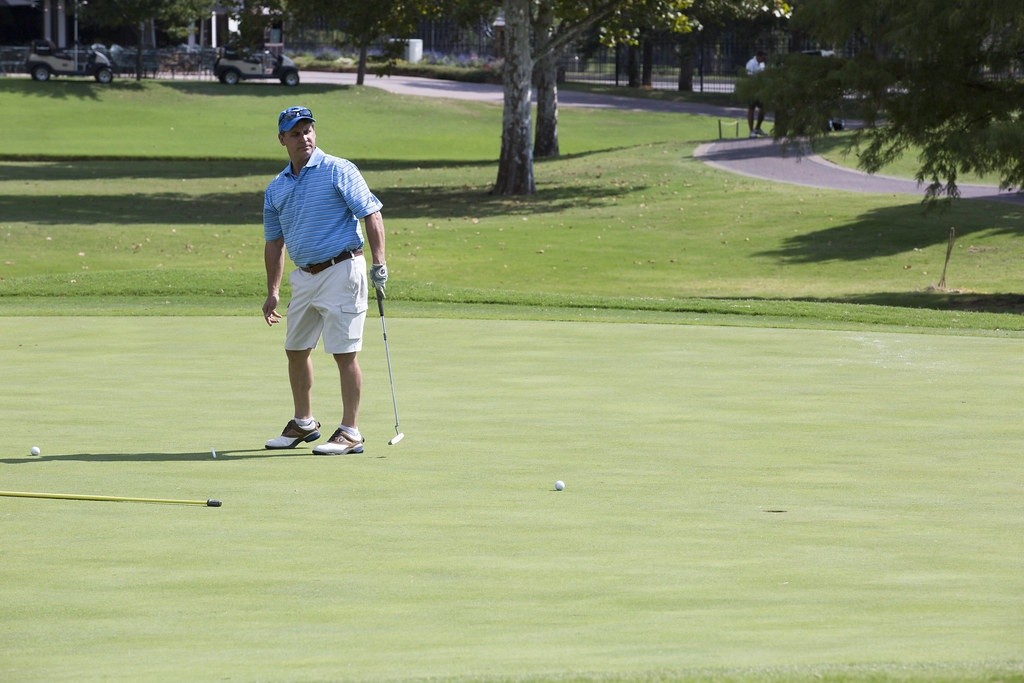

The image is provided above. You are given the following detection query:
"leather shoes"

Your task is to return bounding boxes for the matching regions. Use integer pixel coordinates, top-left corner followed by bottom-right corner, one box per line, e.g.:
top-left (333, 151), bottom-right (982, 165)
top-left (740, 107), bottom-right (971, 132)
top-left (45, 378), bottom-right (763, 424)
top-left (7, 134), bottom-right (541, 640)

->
top-left (265, 420), bottom-right (321, 449)
top-left (312, 429), bottom-right (364, 455)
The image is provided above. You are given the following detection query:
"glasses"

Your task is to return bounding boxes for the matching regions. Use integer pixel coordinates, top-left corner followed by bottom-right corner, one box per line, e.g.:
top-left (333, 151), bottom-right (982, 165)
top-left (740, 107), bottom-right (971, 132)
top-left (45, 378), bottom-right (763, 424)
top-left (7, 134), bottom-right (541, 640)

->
top-left (279, 109), bottom-right (313, 125)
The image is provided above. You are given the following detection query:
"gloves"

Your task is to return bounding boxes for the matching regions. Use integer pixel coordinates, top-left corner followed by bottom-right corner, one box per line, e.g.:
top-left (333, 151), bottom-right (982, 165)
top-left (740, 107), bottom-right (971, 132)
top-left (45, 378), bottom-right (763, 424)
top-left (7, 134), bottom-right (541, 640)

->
top-left (370, 263), bottom-right (387, 298)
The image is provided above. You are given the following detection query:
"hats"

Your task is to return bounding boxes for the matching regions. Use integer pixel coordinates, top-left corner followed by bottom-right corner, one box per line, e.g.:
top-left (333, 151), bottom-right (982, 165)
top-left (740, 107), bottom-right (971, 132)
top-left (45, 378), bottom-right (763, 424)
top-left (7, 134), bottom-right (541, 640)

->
top-left (278, 106), bottom-right (315, 132)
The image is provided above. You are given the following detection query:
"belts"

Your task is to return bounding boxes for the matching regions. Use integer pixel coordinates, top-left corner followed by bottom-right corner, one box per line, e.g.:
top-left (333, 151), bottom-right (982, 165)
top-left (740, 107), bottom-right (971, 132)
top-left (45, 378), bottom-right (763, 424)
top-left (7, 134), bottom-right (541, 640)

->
top-left (300, 248), bottom-right (364, 275)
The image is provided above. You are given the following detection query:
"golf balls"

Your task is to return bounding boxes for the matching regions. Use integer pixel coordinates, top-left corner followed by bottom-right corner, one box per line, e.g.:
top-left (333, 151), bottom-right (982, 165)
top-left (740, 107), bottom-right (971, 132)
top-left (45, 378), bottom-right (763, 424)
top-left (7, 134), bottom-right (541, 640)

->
top-left (31, 446), bottom-right (40, 455)
top-left (556, 481), bottom-right (565, 490)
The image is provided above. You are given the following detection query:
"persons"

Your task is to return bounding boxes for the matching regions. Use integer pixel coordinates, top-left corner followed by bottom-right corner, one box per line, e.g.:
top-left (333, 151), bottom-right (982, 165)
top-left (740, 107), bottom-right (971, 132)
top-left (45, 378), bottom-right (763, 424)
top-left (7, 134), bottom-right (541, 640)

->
top-left (93, 39), bottom-right (124, 67)
top-left (262, 106), bottom-right (388, 455)
top-left (746, 49), bottom-right (768, 138)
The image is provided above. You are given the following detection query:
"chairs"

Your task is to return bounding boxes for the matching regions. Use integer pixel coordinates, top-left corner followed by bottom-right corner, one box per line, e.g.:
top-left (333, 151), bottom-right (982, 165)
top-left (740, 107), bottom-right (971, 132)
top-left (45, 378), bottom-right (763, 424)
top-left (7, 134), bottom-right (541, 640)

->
top-left (0, 42), bottom-right (218, 77)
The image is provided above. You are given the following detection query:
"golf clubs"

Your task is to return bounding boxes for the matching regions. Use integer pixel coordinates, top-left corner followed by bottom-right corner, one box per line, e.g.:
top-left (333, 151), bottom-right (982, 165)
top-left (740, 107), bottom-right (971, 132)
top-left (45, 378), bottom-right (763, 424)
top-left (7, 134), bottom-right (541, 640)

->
top-left (375, 288), bottom-right (405, 445)
